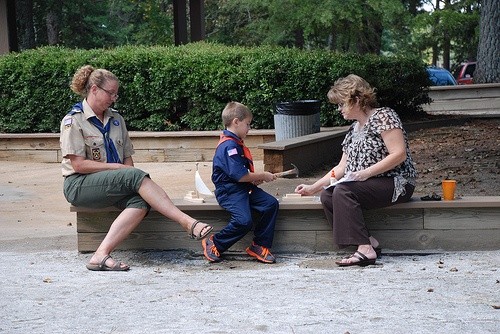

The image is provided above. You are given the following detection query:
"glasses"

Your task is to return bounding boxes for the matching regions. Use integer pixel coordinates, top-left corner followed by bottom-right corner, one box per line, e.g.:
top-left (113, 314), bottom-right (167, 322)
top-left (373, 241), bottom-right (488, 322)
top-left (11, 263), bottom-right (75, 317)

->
top-left (88, 84), bottom-right (119, 101)
top-left (338, 103), bottom-right (344, 106)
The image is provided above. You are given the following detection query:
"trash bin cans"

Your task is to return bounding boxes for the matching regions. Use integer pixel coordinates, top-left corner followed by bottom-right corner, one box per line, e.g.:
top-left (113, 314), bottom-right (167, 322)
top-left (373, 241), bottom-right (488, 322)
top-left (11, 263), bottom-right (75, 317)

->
top-left (273, 99), bottom-right (321, 142)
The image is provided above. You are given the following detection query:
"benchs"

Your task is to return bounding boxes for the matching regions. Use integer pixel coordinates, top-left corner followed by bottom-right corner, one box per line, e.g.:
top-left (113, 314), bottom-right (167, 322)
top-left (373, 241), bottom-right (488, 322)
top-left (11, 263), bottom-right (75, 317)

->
top-left (406, 83), bottom-right (500, 118)
top-left (70, 195), bottom-right (500, 253)
top-left (0, 124), bottom-right (353, 178)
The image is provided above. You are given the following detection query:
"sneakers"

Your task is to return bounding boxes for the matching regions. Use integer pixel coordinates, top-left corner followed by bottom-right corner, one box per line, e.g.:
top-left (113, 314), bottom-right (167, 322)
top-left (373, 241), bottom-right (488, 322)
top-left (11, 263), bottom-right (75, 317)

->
top-left (201, 235), bottom-right (220, 261)
top-left (246, 241), bottom-right (276, 263)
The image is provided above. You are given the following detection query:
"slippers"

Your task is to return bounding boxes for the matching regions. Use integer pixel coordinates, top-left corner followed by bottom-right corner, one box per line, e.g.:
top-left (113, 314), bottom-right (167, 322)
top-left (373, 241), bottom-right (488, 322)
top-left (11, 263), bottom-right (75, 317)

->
top-left (336, 251), bottom-right (376, 266)
top-left (372, 243), bottom-right (383, 257)
top-left (186, 220), bottom-right (213, 240)
top-left (86, 255), bottom-right (130, 271)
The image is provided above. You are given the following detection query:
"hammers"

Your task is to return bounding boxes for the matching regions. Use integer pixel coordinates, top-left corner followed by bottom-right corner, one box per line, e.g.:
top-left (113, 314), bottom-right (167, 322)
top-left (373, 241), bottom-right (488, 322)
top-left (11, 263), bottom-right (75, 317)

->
top-left (273, 162), bottom-right (300, 178)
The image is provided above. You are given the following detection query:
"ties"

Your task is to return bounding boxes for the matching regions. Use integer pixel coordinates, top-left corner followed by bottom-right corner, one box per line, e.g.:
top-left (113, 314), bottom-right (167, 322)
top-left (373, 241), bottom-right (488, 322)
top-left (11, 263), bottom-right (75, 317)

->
top-left (216, 135), bottom-right (255, 173)
top-left (70, 103), bottom-right (122, 163)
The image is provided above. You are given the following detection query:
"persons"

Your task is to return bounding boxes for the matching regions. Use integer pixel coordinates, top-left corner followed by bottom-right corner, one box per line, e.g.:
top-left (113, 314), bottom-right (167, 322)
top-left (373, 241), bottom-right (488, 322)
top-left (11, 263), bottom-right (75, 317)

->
top-left (60, 65), bottom-right (213, 271)
top-left (202, 102), bottom-right (279, 264)
top-left (295, 75), bottom-right (415, 266)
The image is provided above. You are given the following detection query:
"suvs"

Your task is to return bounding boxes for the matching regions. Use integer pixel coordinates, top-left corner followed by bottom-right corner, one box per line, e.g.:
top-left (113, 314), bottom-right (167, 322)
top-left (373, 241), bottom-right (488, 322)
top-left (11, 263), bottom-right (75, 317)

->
top-left (425, 63), bottom-right (458, 86)
top-left (449, 60), bottom-right (477, 85)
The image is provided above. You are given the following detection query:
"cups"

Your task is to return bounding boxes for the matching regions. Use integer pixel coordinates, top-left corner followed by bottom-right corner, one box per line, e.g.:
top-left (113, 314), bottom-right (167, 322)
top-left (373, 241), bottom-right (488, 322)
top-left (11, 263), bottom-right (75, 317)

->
top-left (441, 180), bottom-right (456, 200)
